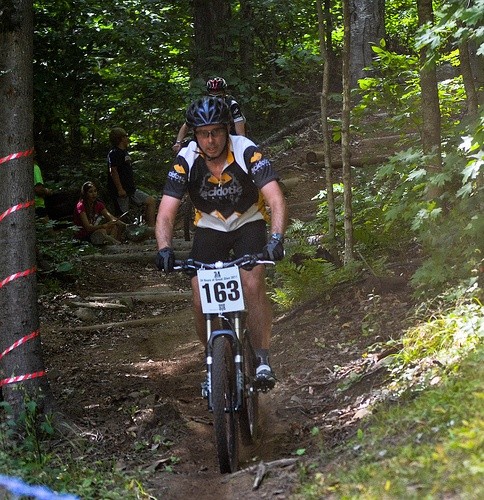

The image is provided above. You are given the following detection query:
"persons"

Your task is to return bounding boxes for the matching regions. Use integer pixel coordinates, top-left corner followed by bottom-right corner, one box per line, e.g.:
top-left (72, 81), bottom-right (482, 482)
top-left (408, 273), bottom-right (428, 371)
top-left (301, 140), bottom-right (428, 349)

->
top-left (152, 97), bottom-right (289, 392)
top-left (172, 77), bottom-right (249, 154)
top-left (73, 181), bottom-right (131, 246)
top-left (33, 142), bottom-right (53, 223)
top-left (107, 128), bottom-right (155, 227)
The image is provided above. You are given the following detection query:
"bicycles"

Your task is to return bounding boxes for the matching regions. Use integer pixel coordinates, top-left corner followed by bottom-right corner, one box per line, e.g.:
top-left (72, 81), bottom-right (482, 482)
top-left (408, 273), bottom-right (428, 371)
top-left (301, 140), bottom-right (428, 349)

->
top-left (151, 250), bottom-right (287, 478)
top-left (177, 135), bottom-right (201, 242)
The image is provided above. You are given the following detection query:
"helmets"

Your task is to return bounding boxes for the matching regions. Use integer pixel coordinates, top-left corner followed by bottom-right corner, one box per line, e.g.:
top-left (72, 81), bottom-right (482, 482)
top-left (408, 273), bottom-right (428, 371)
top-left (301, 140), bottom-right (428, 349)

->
top-left (207, 77), bottom-right (227, 89)
top-left (185, 97), bottom-right (231, 125)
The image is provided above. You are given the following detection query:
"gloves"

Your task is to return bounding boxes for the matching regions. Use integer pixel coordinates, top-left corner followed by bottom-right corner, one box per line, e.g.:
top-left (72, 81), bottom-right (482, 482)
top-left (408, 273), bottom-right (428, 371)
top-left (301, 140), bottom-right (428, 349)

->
top-left (263, 233), bottom-right (285, 262)
top-left (154, 247), bottom-right (176, 273)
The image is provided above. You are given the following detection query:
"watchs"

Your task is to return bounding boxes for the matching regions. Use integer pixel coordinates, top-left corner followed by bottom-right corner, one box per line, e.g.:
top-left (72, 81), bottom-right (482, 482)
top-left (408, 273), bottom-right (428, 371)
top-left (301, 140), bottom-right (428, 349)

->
top-left (270, 233), bottom-right (284, 241)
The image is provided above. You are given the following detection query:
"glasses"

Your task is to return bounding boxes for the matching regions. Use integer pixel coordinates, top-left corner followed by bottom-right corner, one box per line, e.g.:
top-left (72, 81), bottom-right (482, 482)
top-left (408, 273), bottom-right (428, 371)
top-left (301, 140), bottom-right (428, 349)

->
top-left (194, 128), bottom-right (229, 137)
top-left (208, 92), bottom-right (226, 98)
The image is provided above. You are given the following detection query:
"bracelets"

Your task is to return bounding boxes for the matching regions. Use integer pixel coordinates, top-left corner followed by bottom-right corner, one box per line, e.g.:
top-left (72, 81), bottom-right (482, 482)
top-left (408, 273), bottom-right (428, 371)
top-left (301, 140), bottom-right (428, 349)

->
top-left (176, 141), bottom-right (181, 144)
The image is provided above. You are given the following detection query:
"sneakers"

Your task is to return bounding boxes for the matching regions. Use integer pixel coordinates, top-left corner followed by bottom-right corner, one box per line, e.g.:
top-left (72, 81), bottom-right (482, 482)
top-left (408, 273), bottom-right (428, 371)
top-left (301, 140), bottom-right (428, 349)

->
top-left (255, 356), bottom-right (277, 387)
top-left (202, 370), bottom-right (215, 401)
